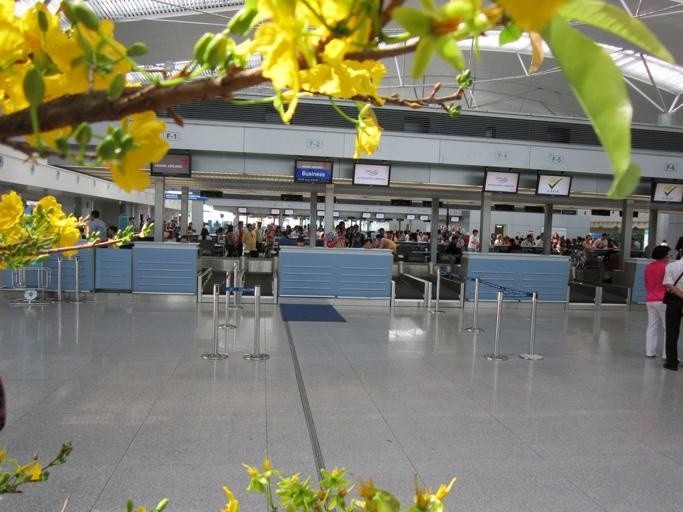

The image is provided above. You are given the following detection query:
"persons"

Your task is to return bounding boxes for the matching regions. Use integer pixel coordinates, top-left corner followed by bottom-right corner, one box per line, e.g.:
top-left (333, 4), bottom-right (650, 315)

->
top-left (489, 230), bottom-right (641, 257)
top-left (660, 239), bottom-right (669, 246)
top-left (662, 236), bottom-right (682, 371)
top-left (644, 245), bottom-right (673, 359)
top-left (316, 221), bottom-right (480, 262)
top-left (76, 211), bottom-right (311, 258)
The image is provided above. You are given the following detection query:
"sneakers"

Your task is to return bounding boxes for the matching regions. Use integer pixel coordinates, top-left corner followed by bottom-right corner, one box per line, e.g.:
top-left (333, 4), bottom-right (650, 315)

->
top-left (645, 353), bottom-right (681, 371)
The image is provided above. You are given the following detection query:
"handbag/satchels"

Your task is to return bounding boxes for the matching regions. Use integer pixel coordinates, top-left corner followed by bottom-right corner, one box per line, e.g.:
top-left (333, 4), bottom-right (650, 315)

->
top-left (662, 291), bottom-right (669, 304)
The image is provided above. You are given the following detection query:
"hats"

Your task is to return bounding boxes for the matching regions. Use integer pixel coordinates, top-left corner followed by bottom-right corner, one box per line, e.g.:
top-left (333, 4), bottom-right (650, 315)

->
top-left (651, 245), bottom-right (671, 260)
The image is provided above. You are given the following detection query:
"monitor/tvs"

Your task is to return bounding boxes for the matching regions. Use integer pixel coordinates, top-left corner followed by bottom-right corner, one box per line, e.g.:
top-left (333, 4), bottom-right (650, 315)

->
top-left (535, 174), bottom-right (573, 197)
top-left (237, 207), bottom-right (247, 215)
top-left (407, 215), bottom-right (416, 219)
top-left (294, 159), bottom-right (333, 184)
top-left (316, 211), bottom-right (324, 216)
top-left (150, 153), bottom-right (191, 177)
top-left (376, 214), bottom-right (385, 219)
top-left (483, 168), bottom-right (521, 194)
top-left (284, 209), bottom-right (293, 215)
top-left (271, 209), bottom-right (280, 214)
top-left (333, 212), bottom-right (339, 217)
top-left (451, 217), bottom-right (459, 222)
top-left (651, 180), bottom-right (683, 205)
top-left (362, 213), bottom-right (371, 219)
top-left (352, 162), bottom-right (391, 187)
top-left (420, 215), bottom-right (429, 220)
